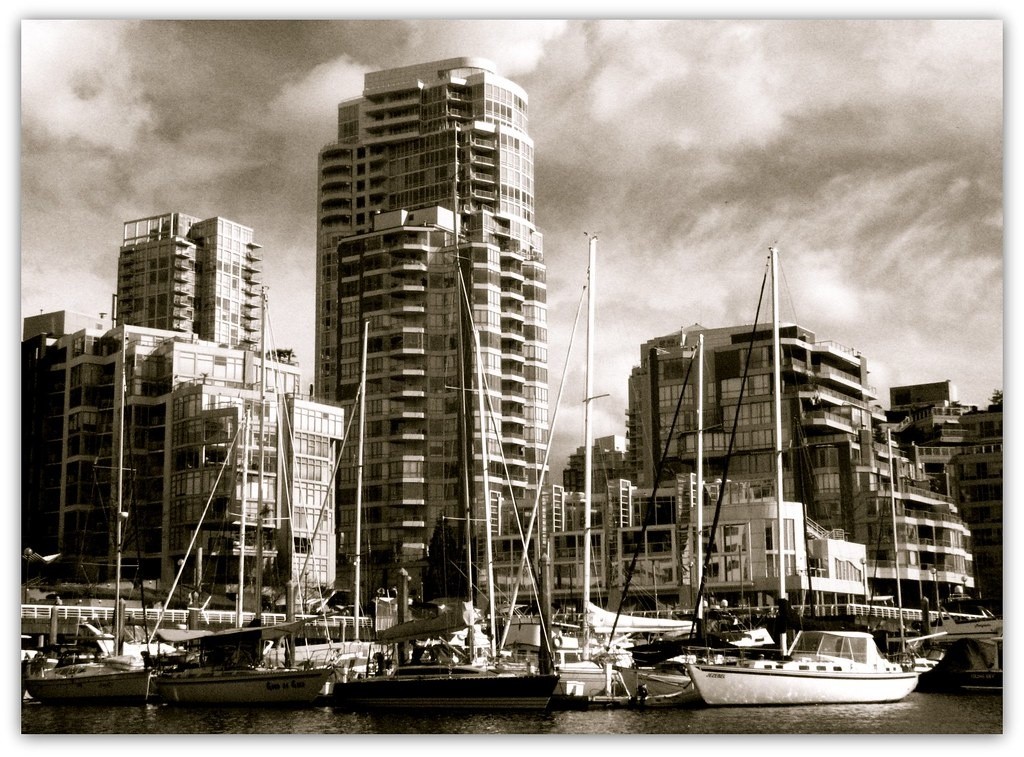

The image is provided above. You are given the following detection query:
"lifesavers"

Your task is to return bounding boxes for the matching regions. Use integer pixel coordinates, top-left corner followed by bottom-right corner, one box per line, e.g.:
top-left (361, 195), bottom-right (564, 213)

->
top-left (552, 636), bottom-right (563, 649)
top-left (900, 653), bottom-right (916, 669)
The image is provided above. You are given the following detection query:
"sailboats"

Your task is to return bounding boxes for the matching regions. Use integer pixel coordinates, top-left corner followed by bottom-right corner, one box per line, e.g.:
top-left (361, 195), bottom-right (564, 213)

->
top-left (23, 233), bottom-right (1001, 708)
